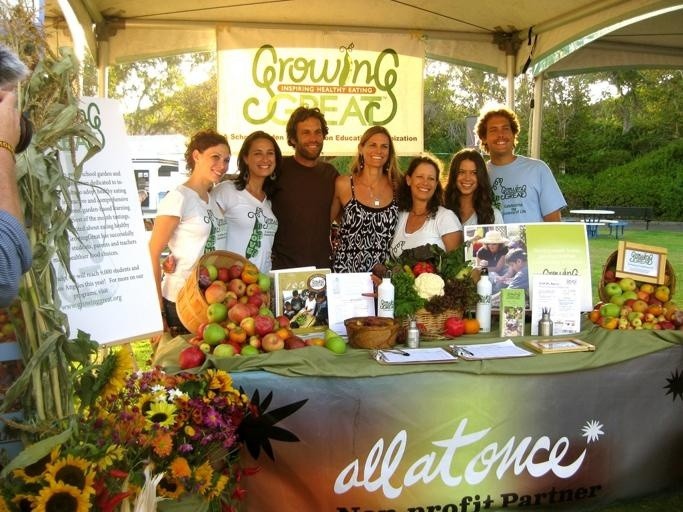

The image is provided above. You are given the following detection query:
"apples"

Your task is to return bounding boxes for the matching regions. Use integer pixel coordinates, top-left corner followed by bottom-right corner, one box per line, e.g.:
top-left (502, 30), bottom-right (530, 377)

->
top-left (589, 270), bottom-right (683, 330)
top-left (189, 260), bottom-right (346, 356)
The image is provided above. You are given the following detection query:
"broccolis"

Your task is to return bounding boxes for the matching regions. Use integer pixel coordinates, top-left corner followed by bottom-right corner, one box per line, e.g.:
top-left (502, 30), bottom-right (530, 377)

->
top-left (413, 272), bottom-right (445, 300)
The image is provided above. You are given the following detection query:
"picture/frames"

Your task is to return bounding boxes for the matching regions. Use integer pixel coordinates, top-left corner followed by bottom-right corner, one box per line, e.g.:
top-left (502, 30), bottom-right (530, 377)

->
top-left (615, 239), bottom-right (669, 286)
top-left (521, 336), bottom-right (598, 356)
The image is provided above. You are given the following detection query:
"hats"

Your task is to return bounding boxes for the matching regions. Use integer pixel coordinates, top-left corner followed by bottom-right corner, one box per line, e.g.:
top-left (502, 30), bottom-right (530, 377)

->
top-left (478, 231), bottom-right (508, 244)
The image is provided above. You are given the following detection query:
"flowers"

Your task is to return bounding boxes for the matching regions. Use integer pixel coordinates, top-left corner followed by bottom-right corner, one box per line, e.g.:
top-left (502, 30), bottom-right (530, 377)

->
top-left (1, 358), bottom-right (310, 512)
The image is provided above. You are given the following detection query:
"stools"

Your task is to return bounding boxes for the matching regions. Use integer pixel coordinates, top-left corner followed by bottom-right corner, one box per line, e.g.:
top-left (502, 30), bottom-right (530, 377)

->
top-left (565, 219), bottom-right (628, 241)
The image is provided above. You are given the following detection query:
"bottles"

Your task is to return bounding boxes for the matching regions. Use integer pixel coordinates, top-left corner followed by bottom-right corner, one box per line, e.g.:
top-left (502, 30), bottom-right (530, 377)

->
top-left (475, 267), bottom-right (494, 333)
top-left (538, 313), bottom-right (554, 337)
top-left (376, 271), bottom-right (394, 318)
top-left (406, 319), bottom-right (420, 348)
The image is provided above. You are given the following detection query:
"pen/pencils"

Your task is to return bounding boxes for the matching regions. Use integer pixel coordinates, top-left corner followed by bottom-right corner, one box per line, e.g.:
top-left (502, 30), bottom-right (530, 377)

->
top-left (461, 347), bottom-right (474, 356)
top-left (382, 348), bottom-right (410, 356)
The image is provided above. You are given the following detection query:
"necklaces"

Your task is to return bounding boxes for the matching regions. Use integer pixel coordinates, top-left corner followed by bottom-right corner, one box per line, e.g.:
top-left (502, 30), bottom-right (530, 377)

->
top-left (356, 175), bottom-right (392, 207)
top-left (408, 209), bottom-right (431, 217)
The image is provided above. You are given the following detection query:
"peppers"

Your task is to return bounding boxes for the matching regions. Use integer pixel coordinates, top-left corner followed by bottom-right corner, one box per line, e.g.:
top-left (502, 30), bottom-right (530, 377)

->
top-left (413, 260), bottom-right (433, 276)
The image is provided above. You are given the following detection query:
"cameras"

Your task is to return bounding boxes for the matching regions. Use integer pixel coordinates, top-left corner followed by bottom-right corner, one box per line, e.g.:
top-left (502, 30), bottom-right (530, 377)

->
top-left (15, 115), bottom-right (32, 153)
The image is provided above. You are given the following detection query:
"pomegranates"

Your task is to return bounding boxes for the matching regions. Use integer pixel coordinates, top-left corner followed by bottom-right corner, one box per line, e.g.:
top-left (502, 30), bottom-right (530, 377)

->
top-left (179, 344), bottom-right (204, 369)
top-left (444, 316), bottom-right (465, 335)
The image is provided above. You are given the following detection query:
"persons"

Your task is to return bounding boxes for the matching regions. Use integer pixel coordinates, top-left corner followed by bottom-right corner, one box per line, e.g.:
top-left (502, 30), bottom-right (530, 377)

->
top-left (443, 145), bottom-right (506, 227)
top-left (263, 104), bottom-right (344, 275)
top-left (149, 130), bottom-right (231, 338)
top-left (162, 129), bottom-right (279, 277)
top-left (327, 126), bottom-right (408, 273)
top-left (464, 229), bottom-right (529, 309)
top-left (280, 287), bottom-right (327, 328)
top-left (388, 155), bottom-right (463, 272)
top-left (471, 99), bottom-right (565, 221)
top-left (136, 187), bottom-right (151, 207)
top-left (0, 88), bottom-right (37, 305)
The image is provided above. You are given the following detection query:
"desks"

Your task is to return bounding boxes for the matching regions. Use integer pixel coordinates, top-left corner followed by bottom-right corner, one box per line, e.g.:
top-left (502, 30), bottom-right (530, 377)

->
top-left (150, 330), bottom-right (682, 512)
top-left (568, 209), bottom-right (616, 235)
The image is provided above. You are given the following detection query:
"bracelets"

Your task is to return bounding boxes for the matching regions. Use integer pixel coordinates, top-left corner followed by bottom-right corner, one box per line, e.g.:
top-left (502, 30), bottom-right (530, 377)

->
top-left (0, 142), bottom-right (20, 165)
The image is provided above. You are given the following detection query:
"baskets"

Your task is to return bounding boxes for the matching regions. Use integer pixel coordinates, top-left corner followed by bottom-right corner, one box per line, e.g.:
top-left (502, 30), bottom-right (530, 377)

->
top-left (176, 250), bottom-right (270, 335)
top-left (344, 316), bottom-right (399, 349)
top-left (403, 306), bottom-right (463, 341)
top-left (598, 251), bottom-right (675, 304)
